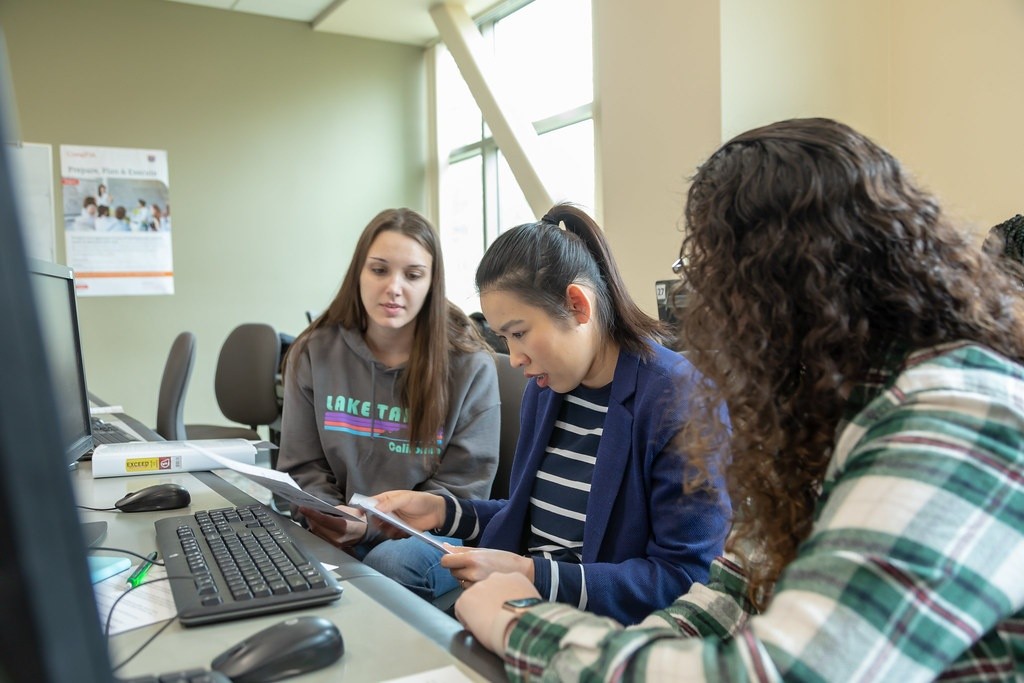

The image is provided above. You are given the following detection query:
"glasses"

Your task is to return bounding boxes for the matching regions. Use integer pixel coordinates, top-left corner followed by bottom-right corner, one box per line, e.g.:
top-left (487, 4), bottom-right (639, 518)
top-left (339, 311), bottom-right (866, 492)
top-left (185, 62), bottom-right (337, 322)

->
top-left (671, 255), bottom-right (695, 274)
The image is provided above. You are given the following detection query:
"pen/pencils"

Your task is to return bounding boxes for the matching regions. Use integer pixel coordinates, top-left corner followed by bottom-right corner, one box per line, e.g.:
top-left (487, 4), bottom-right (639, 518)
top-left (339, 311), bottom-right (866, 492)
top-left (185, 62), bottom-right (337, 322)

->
top-left (125, 551), bottom-right (158, 590)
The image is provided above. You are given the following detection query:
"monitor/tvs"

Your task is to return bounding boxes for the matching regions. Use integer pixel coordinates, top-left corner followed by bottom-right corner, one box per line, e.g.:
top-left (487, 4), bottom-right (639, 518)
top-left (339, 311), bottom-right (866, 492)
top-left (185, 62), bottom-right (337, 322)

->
top-left (0, 92), bottom-right (113, 683)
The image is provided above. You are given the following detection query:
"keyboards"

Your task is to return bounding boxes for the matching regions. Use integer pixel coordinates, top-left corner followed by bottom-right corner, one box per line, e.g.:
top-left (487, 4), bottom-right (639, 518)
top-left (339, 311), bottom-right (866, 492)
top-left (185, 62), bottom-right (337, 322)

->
top-left (112, 666), bottom-right (232, 683)
top-left (76, 423), bottom-right (140, 460)
top-left (154, 502), bottom-right (343, 629)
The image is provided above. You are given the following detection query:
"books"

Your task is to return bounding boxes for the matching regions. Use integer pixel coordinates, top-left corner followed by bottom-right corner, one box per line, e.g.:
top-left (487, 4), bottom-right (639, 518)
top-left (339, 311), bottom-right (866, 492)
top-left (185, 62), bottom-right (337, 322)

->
top-left (92, 439), bottom-right (257, 479)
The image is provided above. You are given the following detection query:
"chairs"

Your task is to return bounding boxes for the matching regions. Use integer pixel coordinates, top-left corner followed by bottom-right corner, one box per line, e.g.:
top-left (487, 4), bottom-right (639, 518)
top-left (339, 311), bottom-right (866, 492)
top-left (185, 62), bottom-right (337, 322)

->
top-left (152, 323), bottom-right (279, 439)
top-left (492, 350), bottom-right (536, 502)
top-left (156, 332), bottom-right (196, 440)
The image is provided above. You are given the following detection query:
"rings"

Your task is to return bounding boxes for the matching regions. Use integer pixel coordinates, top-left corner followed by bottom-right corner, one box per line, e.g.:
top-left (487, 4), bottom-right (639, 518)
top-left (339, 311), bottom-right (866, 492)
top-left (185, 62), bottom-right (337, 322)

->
top-left (459, 579), bottom-right (464, 589)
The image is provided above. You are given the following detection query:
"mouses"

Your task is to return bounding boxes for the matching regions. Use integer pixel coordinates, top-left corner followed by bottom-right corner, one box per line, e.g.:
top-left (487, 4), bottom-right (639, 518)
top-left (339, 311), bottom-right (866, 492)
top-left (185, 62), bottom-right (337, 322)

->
top-left (211, 616), bottom-right (345, 682)
top-left (115, 483), bottom-right (191, 513)
top-left (91, 417), bottom-right (103, 424)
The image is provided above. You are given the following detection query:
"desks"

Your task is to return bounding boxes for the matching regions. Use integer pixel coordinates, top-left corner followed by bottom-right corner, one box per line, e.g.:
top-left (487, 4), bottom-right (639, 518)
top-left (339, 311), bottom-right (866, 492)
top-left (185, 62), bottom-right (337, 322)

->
top-left (70, 392), bottom-right (508, 683)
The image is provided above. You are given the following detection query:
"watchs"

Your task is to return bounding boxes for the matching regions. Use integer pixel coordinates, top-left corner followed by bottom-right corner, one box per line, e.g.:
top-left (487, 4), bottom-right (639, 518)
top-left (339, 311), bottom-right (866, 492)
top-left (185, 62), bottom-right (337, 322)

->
top-left (492, 597), bottom-right (544, 660)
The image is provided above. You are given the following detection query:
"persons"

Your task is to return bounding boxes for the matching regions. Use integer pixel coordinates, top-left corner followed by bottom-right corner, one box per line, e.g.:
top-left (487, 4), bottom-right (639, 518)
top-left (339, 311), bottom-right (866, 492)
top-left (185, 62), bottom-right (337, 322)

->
top-left (72, 184), bottom-right (171, 233)
top-left (272, 118), bottom-right (1024, 683)
